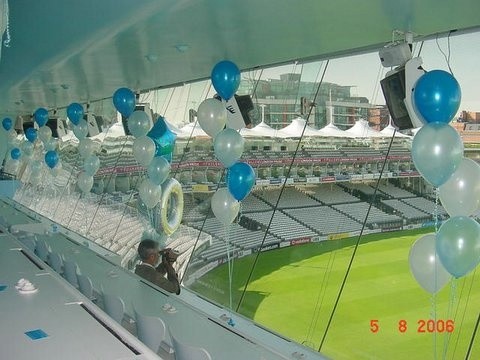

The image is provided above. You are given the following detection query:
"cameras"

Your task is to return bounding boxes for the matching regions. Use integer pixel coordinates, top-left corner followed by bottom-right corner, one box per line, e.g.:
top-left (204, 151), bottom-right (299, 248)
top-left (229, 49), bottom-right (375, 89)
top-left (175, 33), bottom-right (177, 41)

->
top-left (159, 248), bottom-right (180, 262)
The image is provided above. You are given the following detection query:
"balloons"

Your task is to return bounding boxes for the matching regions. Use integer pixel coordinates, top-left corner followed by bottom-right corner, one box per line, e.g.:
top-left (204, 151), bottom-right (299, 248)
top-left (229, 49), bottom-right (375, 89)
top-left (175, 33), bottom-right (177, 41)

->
top-left (214, 129), bottom-right (243, 168)
top-left (438, 157), bottom-right (480, 218)
top-left (1, 108), bottom-right (61, 185)
top-left (435, 215), bottom-right (480, 279)
top-left (147, 156), bottom-right (169, 184)
top-left (412, 121), bottom-right (464, 188)
top-left (413, 69), bottom-right (462, 127)
top-left (211, 60), bottom-right (241, 102)
top-left (128, 110), bottom-right (153, 137)
top-left (139, 180), bottom-right (162, 208)
top-left (67, 103), bottom-right (100, 193)
top-left (211, 188), bottom-right (240, 224)
top-left (410, 233), bottom-right (451, 295)
top-left (197, 98), bottom-right (227, 137)
top-left (132, 136), bottom-right (156, 165)
top-left (113, 88), bottom-right (136, 117)
top-left (225, 162), bottom-right (256, 202)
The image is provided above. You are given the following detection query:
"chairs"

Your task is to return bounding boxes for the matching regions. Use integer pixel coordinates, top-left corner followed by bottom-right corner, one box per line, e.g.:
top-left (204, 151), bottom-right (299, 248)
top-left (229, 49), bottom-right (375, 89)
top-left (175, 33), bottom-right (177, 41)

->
top-left (18, 224), bottom-right (211, 360)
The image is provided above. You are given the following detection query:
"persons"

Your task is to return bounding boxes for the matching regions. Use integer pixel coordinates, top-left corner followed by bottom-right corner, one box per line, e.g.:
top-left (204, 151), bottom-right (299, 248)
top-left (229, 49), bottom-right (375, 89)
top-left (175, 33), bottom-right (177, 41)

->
top-left (134, 239), bottom-right (179, 293)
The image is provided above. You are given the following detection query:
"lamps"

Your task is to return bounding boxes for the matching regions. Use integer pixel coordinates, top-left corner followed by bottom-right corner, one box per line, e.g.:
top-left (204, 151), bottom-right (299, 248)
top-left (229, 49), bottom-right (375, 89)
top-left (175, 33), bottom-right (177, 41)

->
top-left (15, 118), bottom-right (68, 142)
top-left (119, 91), bottom-right (157, 135)
top-left (83, 108), bottom-right (109, 137)
top-left (376, 30), bottom-right (429, 132)
top-left (211, 68), bottom-right (271, 131)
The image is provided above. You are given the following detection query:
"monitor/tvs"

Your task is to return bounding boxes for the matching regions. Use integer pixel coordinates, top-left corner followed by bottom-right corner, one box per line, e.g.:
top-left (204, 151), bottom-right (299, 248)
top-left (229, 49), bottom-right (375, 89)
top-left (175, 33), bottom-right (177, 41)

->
top-left (83, 114), bottom-right (103, 137)
top-left (45, 117), bottom-right (67, 137)
top-left (211, 93), bottom-right (254, 131)
top-left (380, 66), bottom-right (430, 130)
top-left (23, 121), bottom-right (39, 134)
top-left (120, 103), bottom-right (155, 136)
